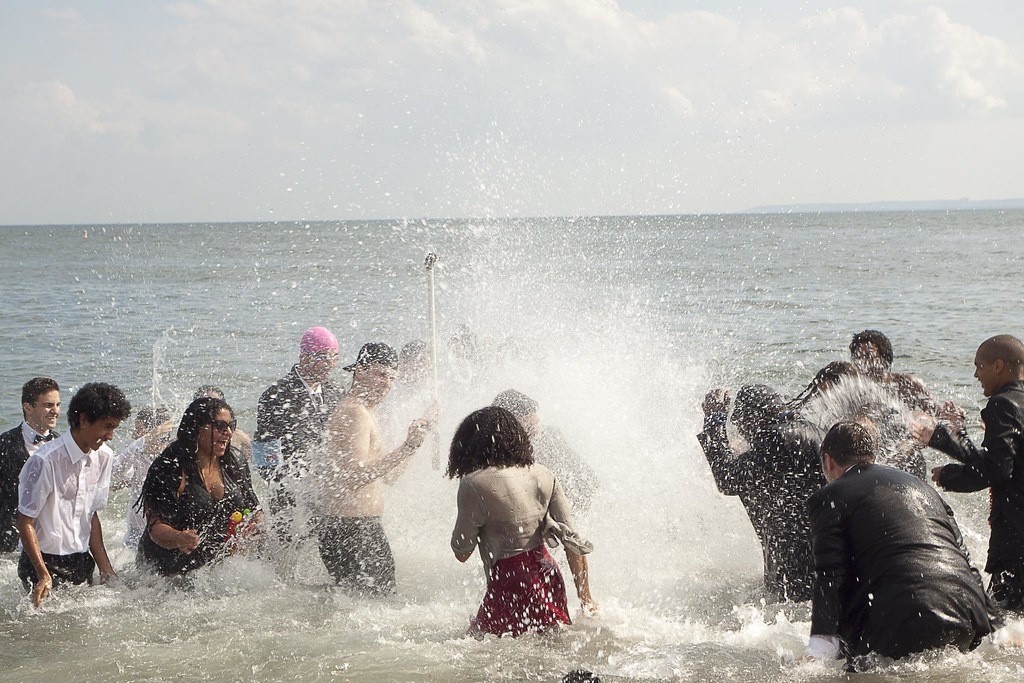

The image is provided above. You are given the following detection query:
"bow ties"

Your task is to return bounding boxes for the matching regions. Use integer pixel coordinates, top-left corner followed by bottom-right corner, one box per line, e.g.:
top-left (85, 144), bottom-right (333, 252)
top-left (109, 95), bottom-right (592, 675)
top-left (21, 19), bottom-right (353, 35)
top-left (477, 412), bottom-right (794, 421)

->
top-left (33, 429), bottom-right (53, 444)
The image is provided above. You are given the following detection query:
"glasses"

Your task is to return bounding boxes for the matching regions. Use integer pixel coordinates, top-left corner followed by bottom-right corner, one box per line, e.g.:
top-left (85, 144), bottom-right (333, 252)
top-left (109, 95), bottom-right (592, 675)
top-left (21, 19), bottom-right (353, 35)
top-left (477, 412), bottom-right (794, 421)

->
top-left (206, 420), bottom-right (237, 434)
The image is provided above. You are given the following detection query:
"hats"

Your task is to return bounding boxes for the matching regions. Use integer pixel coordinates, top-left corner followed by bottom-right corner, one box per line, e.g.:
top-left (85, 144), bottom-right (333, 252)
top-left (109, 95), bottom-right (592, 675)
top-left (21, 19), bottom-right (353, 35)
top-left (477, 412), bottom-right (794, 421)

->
top-left (342, 343), bottom-right (398, 373)
top-left (299, 326), bottom-right (339, 353)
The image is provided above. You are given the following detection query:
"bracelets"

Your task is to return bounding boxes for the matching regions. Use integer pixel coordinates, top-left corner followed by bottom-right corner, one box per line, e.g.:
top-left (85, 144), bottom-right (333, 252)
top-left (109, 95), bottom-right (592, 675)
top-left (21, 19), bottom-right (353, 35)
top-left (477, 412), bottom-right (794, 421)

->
top-left (403, 440), bottom-right (417, 453)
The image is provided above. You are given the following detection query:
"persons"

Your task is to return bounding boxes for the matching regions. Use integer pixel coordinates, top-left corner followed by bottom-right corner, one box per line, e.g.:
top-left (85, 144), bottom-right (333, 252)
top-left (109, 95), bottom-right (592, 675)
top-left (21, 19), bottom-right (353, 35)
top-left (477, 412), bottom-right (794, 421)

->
top-left (806, 418), bottom-right (1007, 672)
top-left (448, 406), bottom-right (602, 640)
top-left (697, 330), bottom-right (972, 627)
top-left (907, 333), bottom-right (1023, 623)
top-left (17, 382), bottom-right (133, 609)
top-left (105, 323), bottom-right (598, 606)
top-left (0, 375), bottom-right (64, 560)
